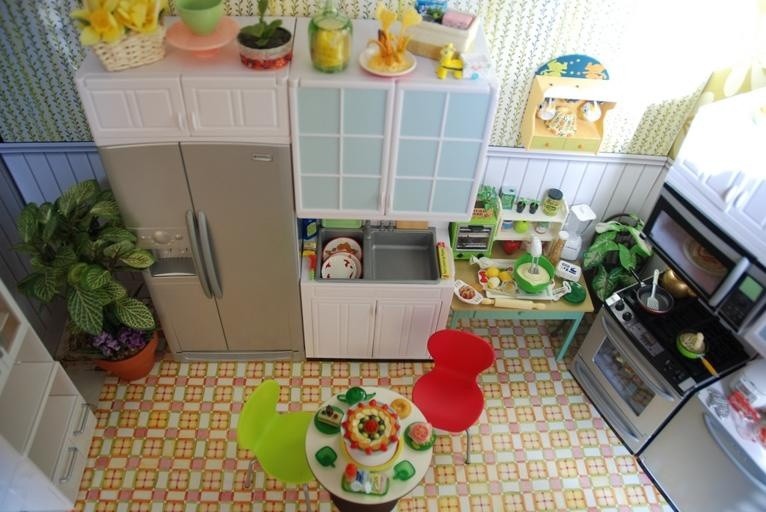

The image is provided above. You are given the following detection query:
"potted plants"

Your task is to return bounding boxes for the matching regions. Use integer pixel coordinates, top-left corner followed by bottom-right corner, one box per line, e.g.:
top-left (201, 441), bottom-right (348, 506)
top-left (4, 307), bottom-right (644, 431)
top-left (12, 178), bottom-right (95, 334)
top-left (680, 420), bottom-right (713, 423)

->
top-left (8, 178), bottom-right (159, 382)
top-left (581, 220), bottom-right (653, 313)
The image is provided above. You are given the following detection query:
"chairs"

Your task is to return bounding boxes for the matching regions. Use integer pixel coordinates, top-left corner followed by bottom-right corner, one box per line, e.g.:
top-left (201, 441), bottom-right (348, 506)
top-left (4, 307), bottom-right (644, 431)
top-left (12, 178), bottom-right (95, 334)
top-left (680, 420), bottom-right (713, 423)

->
top-left (236, 380), bottom-right (318, 512)
top-left (411, 327), bottom-right (496, 465)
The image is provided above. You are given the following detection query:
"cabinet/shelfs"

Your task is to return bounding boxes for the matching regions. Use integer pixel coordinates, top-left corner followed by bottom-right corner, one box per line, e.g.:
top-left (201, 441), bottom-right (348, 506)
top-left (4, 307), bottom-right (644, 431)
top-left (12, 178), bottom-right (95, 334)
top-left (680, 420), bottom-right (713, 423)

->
top-left (290, 18), bottom-right (498, 224)
top-left (300, 284), bottom-right (452, 360)
top-left (75, 17), bottom-right (296, 142)
top-left (492, 199), bottom-right (567, 255)
top-left (0, 277), bottom-right (97, 512)
top-left (519, 73), bottom-right (617, 156)
top-left (674, 88), bottom-right (766, 249)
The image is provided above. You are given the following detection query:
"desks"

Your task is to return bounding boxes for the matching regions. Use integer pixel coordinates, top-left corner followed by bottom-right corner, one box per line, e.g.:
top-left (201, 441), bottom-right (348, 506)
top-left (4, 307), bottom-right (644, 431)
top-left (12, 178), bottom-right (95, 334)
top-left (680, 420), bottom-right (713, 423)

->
top-left (305, 386), bottom-right (434, 512)
top-left (451, 258), bottom-right (594, 362)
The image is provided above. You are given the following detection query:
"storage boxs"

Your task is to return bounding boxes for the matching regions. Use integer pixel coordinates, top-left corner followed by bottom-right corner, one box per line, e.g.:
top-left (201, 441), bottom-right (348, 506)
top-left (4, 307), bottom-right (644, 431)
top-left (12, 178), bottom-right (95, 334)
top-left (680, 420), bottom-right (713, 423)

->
top-left (406, 11), bottom-right (478, 63)
